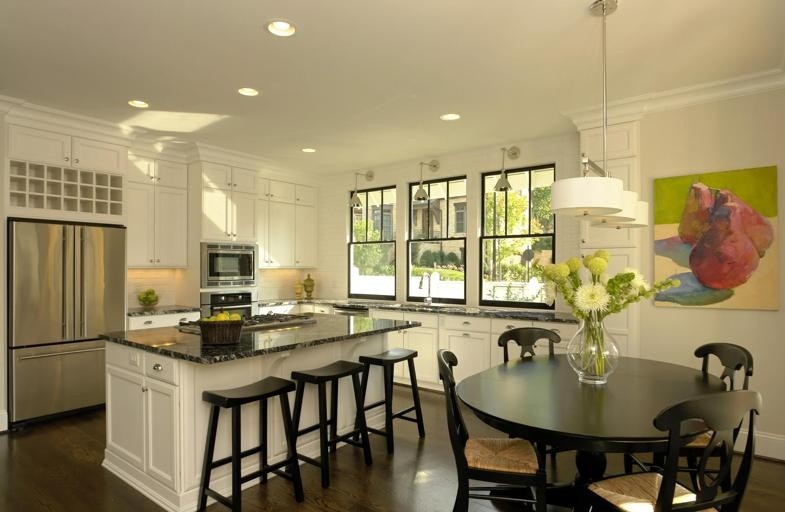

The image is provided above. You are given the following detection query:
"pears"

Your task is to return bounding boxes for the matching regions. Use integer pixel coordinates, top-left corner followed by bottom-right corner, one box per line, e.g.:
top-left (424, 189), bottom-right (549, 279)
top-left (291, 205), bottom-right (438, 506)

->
top-left (201, 311), bottom-right (242, 323)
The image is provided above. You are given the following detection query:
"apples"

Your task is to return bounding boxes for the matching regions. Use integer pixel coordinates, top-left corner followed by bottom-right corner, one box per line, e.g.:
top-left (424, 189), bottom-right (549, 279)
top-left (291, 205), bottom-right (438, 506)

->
top-left (137, 288), bottom-right (158, 305)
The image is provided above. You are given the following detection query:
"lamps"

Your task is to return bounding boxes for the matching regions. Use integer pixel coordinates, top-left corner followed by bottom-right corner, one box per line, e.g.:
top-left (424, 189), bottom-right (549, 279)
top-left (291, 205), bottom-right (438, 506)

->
top-left (261, 16), bottom-right (298, 43)
top-left (346, 170), bottom-right (375, 210)
top-left (548, 2), bottom-right (651, 232)
top-left (493, 146), bottom-right (521, 194)
top-left (413, 159), bottom-right (439, 202)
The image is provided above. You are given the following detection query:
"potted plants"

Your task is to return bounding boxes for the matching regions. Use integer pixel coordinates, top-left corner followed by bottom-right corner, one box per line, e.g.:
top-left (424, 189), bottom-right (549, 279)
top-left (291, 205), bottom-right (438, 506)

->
top-left (136, 286), bottom-right (161, 312)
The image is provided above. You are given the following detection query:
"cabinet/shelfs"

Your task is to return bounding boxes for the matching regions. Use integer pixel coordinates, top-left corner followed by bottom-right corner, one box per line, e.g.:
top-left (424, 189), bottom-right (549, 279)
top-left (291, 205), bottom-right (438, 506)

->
top-left (437, 311), bottom-right (492, 393)
top-left (125, 157), bottom-right (193, 272)
top-left (576, 158), bottom-right (635, 249)
top-left (259, 303), bottom-right (301, 320)
top-left (571, 249), bottom-right (634, 358)
top-left (253, 172), bottom-right (297, 271)
top-left (295, 184), bottom-right (320, 269)
top-left (578, 120), bottom-right (638, 164)
top-left (369, 308), bottom-right (440, 394)
top-left (489, 316), bottom-right (579, 369)
top-left (128, 304), bottom-right (199, 334)
top-left (102, 310), bottom-right (423, 510)
top-left (199, 160), bottom-right (256, 246)
top-left (298, 300), bottom-right (333, 317)
top-left (6, 121), bottom-right (127, 226)
top-left (100, 333), bottom-right (179, 511)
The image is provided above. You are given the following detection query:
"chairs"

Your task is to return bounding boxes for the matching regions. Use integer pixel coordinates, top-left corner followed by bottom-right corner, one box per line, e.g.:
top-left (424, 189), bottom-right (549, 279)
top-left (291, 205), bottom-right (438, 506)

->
top-left (653, 340), bottom-right (756, 492)
top-left (498, 323), bottom-right (563, 473)
top-left (437, 349), bottom-right (547, 511)
top-left (578, 388), bottom-right (764, 512)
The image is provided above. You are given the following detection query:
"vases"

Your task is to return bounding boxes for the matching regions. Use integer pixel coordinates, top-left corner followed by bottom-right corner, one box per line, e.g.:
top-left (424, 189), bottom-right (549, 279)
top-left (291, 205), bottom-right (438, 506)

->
top-left (564, 316), bottom-right (621, 387)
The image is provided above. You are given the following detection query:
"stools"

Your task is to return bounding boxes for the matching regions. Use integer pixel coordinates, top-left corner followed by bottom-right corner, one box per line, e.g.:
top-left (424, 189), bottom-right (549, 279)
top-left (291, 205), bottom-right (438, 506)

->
top-left (352, 344), bottom-right (425, 455)
top-left (286, 354), bottom-right (374, 489)
top-left (196, 373), bottom-right (305, 511)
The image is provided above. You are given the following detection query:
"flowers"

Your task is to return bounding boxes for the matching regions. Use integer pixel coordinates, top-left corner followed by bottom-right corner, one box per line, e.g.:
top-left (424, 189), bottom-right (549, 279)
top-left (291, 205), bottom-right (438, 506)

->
top-left (532, 246), bottom-right (682, 374)
